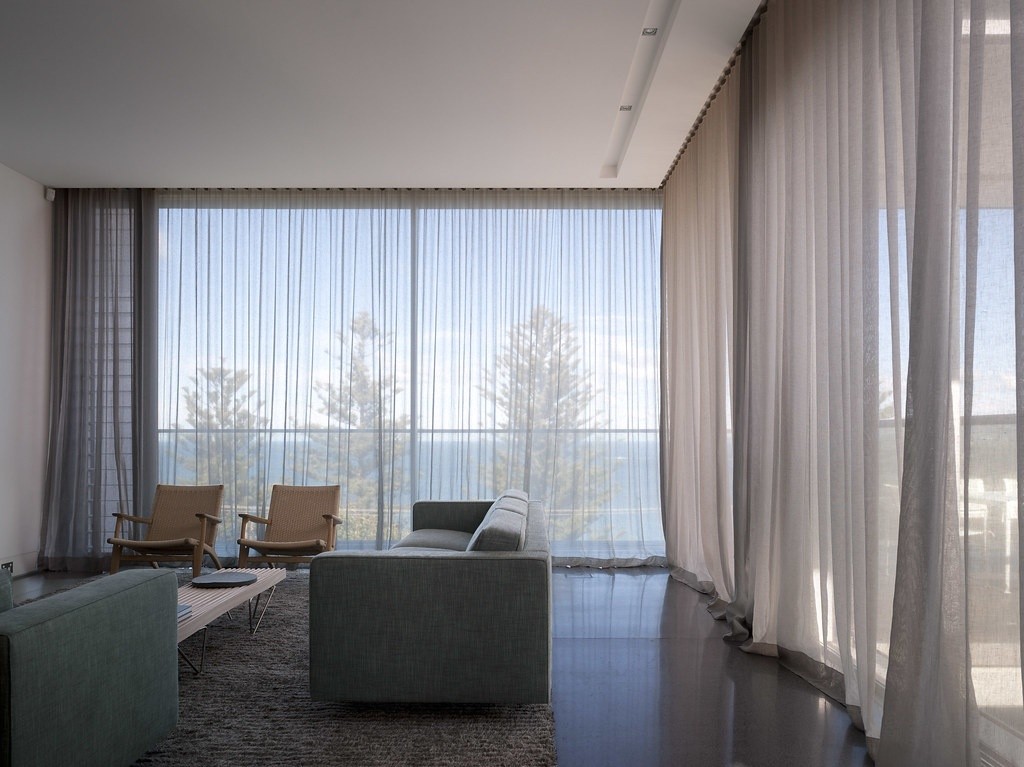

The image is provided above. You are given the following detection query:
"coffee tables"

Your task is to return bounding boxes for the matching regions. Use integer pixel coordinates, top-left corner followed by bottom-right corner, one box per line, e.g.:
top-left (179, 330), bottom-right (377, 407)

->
top-left (178, 567), bottom-right (287, 674)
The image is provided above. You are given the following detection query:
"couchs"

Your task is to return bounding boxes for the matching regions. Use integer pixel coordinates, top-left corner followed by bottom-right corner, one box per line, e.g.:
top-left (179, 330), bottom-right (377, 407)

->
top-left (310, 500), bottom-right (553, 705)
top-left (0, 568), bottom-right (180, 767)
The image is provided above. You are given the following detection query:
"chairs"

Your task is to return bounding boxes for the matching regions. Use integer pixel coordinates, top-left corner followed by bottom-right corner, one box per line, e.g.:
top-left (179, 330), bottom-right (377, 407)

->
top-left (237, 485), bottom-right (343, 568)
top-left (107, 484), bottom-right (224, 579)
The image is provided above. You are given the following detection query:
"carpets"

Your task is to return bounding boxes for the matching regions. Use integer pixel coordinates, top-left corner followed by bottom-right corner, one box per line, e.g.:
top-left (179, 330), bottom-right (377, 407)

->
top-left (16, 568), bottom-right (558, 767)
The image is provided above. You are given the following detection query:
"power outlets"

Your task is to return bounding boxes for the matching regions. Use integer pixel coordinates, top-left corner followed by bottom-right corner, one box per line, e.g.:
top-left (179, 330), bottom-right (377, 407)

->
top-left (1, 562), bottom-right (13, 573)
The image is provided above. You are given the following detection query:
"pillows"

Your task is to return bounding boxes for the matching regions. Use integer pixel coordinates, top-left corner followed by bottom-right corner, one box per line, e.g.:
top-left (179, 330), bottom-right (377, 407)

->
top-left (466, 489), bottom-right (529, 552)
